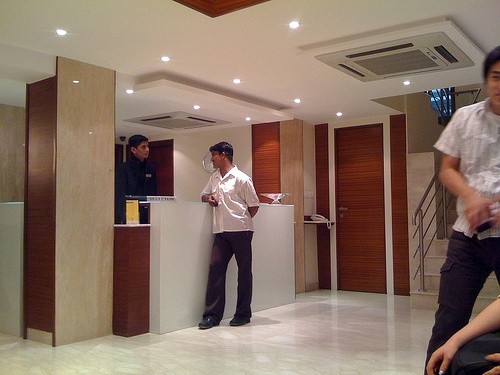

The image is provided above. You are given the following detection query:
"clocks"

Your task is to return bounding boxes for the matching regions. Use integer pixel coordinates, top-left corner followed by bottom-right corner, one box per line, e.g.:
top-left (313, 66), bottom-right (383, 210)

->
top-left (203, 153), bottom-right (218, 173)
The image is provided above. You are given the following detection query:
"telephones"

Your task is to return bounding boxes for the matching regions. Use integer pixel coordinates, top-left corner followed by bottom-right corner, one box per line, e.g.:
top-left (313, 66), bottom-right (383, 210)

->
top-left (310, 214), bottom-right (328, 221)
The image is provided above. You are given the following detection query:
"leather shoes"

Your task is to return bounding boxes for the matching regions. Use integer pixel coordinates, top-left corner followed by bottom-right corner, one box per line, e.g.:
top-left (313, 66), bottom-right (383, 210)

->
top-left (230, 317), bottom-right (250, 325)
top-left (198, 317), bottom-right (219, 329)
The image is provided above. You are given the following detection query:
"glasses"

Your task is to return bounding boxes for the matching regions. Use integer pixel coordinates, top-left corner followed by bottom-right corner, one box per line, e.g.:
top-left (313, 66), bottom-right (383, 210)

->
top-left (211, 153), bottom-right (220, 158)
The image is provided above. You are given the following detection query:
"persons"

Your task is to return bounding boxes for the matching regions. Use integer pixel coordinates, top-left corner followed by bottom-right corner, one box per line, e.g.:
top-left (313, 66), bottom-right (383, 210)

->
top-left (198, 141), bottom-right (261, 330)
top-left (423, 46), bottom-right (500, 375)
top-left (115, 134), bottom-right (160, 226)
top-left (426, 285), bottom-right (500, 375)
